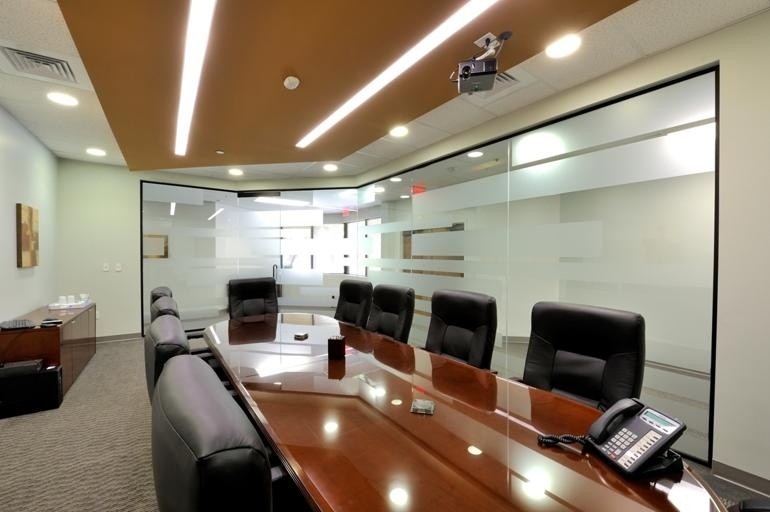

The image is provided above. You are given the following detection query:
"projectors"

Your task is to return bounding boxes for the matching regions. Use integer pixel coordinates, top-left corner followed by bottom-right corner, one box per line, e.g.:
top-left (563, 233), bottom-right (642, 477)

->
top-left (457, 58), bottom-right (498, 94)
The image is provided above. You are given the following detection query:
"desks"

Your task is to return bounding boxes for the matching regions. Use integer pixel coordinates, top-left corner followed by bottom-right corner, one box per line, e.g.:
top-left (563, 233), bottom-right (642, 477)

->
top-left (204, 312), bottom-right (727, 512)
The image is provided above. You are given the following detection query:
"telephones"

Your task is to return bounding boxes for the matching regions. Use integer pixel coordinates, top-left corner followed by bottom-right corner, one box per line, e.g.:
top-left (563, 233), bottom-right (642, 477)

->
top-left (585, 397), bottom-right (686, 476)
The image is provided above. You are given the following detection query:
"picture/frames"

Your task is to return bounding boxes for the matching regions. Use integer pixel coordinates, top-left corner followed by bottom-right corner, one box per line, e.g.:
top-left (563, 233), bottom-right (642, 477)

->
top-left (143, 234), bottom-right (168, 258)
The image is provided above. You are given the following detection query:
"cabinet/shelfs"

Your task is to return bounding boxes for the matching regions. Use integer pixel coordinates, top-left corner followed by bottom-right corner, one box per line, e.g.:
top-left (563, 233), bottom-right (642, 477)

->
top-left (0, 302), bottom-right (96, 396)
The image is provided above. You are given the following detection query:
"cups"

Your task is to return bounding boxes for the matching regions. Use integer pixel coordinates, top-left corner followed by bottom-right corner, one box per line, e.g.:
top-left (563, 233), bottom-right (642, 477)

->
top-left (58, 295), bottom-right (66, 305)
top-left (67, 294), bottom-right (75, 305)
top-left (79, 293), bottom-right (89, 303)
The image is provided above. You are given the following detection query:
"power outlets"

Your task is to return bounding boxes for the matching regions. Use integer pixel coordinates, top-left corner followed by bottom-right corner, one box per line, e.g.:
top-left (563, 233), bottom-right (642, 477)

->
top-left (473, 32), bottom-right (500, 50)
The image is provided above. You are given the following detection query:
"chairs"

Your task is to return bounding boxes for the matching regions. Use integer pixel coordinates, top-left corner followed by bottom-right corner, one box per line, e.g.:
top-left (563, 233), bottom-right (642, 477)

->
top-left (508, 301), bottom-right (645, 413)
top-left (333, 279), bottom-right (373, 326)
top-left (366, 284), bottom-right (414, 343)
top-left (421, 290), bottom-right (498, 375)
top-left (145, 286), bottom-right (313, 512)
top-left (228, 277), bottom-right (279, 319)
